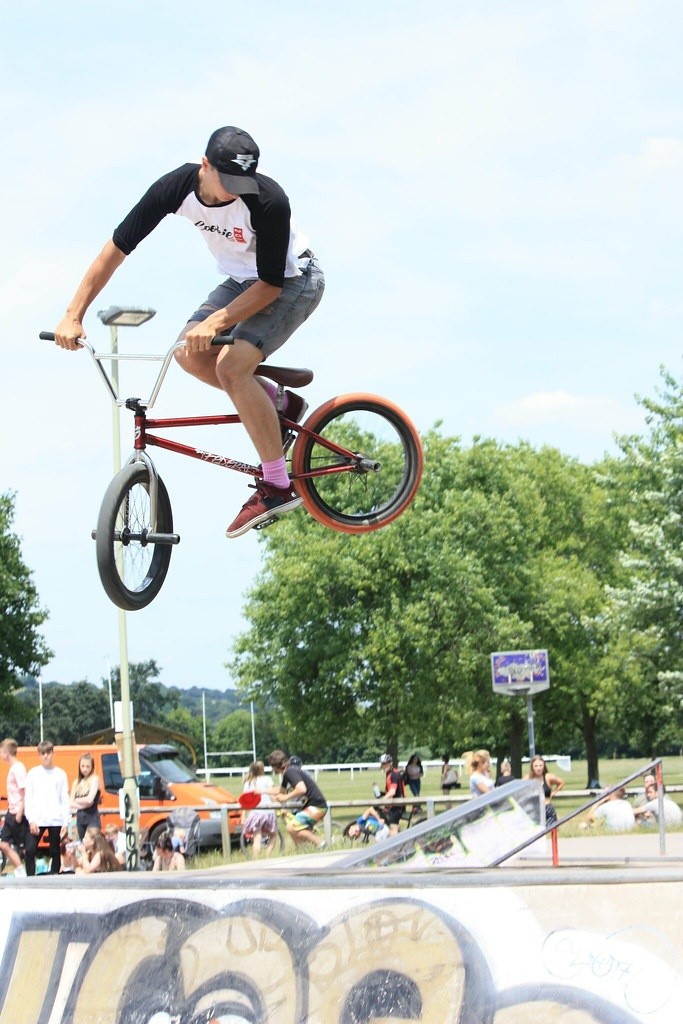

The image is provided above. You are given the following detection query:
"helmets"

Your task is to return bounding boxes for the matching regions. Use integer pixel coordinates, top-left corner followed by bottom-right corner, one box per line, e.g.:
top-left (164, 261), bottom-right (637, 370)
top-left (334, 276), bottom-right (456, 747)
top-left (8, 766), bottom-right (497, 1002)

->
top-left (379, 754), bottom-right (393, 764)
top-left (290, 756), bottom-right (302, 767)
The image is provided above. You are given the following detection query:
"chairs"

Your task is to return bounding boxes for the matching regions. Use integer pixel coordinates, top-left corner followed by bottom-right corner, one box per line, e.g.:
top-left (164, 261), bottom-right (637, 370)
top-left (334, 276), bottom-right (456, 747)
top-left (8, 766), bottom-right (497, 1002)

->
top-left (142, 806), bottom-right (203, 865)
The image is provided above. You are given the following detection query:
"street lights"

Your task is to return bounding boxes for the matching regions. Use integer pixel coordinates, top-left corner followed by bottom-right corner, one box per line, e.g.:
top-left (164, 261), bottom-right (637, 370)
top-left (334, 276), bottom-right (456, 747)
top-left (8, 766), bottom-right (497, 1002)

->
top-left (90, 302), bottom-right (160, 874)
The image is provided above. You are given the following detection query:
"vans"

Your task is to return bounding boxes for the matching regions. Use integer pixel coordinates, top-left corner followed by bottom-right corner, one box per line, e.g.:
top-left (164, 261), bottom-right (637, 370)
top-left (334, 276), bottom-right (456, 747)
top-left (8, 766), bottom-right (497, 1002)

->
top-left (0, 743), bottom-right (271, 854)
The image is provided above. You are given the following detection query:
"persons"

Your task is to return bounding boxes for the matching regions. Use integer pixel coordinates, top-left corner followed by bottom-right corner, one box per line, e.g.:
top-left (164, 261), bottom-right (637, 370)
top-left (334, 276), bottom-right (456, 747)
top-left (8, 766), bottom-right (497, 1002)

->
top-left (348, 806), bottom-right (390, 844)
top-left (635, 775), bottom-right (656, 809)
top-left (377, 754), bottom-right (406, 836)
top-left (106, 825), bottom-right (127, 871)
top-left (269, 750), bottom-right (327, 850)
top-left (441, 755), bottom-right (457, 810)
top-left (470, 749), bottom-right (495, 800)
top-left (241, 760), bottom-right (277, 859)
top-left (522, 756), bottom-right (564, 829)
top-left (589, 787), bottom-right (634, 832)
top-left (496, 762), bottom-right (515, 787)
top-left (151, 837), bottom-right (185, 872)
top-left (53, 125), bottom-right (325, 539)
top-left (0, 738), bottom-right (26, 879)
top-left (71, 754), bottom-right (101, 843)
top-left (405, 755), bottom-right (423, 813)
top-left (632, 783), bottom-right (683, 827)
top-left (74, 826), bottom-right (120, 876)
top-left (24, 741), bottom-right (69, 876)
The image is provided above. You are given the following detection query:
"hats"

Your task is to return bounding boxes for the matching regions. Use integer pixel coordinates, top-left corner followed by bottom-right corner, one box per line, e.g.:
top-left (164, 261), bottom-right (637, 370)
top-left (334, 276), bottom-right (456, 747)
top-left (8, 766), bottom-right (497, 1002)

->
top-left (206, 126), bottom-right (260, 196)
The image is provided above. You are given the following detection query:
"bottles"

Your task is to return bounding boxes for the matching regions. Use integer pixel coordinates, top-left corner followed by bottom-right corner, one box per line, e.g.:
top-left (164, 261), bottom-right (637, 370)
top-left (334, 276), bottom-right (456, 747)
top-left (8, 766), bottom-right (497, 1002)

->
top-left (36, 859), bottom-right (47, 875)
top-left (373, 783), bottom-right (380, 797)
top-left (65, 840), bottom-right (82, 850)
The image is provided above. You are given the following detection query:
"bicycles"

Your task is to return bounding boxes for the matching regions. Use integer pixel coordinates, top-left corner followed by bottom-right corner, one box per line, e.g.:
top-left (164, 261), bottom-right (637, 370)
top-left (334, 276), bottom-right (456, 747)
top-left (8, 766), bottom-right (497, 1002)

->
top-left (36, 306), bottom-right (427, 611)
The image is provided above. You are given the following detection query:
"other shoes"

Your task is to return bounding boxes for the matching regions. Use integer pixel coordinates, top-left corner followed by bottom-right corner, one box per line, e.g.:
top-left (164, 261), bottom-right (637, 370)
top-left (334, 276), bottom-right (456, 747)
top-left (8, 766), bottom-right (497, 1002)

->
top-left (6, 865), bottom-right (27, 879)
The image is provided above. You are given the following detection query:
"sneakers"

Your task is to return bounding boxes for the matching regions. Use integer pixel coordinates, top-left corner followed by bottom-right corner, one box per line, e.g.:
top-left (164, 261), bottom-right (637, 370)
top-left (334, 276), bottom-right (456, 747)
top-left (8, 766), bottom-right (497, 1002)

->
top-left (223, 478), bottom-right (305, 538)
top-left (280, 388), bottom-right (308, 443)
